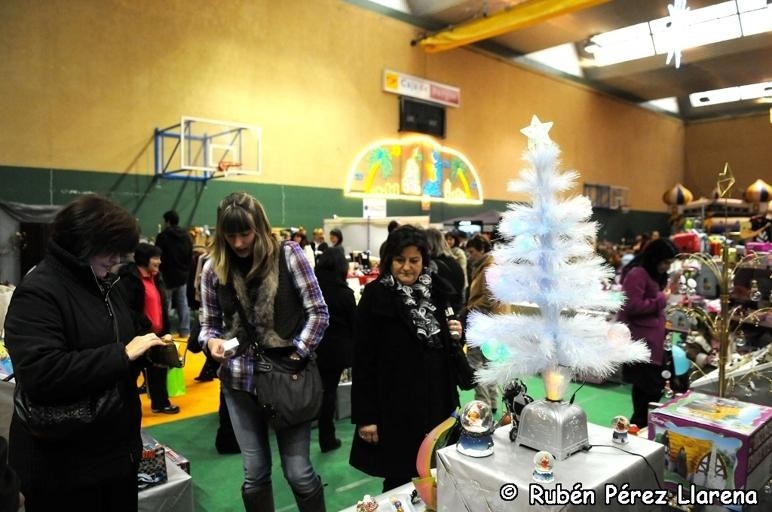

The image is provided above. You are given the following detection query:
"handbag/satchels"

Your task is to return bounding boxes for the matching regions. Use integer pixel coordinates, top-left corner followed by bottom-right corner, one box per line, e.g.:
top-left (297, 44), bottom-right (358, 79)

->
top-left (439, 293), bottom-right (479, 390)
top-left (12, 374), bottom-right (131, 442)
top-left (251, 343), bottom-right (325, 428)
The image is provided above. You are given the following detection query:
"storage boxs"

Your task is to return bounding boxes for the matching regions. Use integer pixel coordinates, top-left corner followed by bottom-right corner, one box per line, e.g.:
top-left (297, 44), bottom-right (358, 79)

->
top-left (138, 431), bottom-right (168, 492)
top-left (159, 444), bottom-right (190, 476)
top-left (648, 389), bottom-right (772, 512)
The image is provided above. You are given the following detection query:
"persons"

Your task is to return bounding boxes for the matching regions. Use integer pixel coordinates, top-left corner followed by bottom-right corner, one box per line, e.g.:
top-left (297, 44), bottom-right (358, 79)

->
top-left (117, 240), bottom-right (181, 414)
top-left (153, 209), bottom-right (195, 340)
top-left (1, 192), bottom-right (176, 512)
top-left (185, 187), bottom-right (679, 512)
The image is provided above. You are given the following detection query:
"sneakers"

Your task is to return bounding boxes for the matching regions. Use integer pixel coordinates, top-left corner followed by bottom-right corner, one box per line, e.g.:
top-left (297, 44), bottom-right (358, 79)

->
top-left (319, 438), bottom-right (342, 453)
top-left (152, 406), bottom-right (180, 414)
top-left (194, 375), bottom-right (214, 381)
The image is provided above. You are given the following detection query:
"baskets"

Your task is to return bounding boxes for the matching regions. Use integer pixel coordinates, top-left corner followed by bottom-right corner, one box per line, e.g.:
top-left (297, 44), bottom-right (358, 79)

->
top-left (136, 342), bottom-right (182, 371)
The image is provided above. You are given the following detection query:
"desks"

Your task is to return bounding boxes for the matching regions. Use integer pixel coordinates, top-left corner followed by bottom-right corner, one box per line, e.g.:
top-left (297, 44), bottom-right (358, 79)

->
top-left (435, 420), bottom-right (664, 512)
top-left (338, 468), bottom-right (437, 512)
top-left (138, 455), bottom-right (193, 511)
